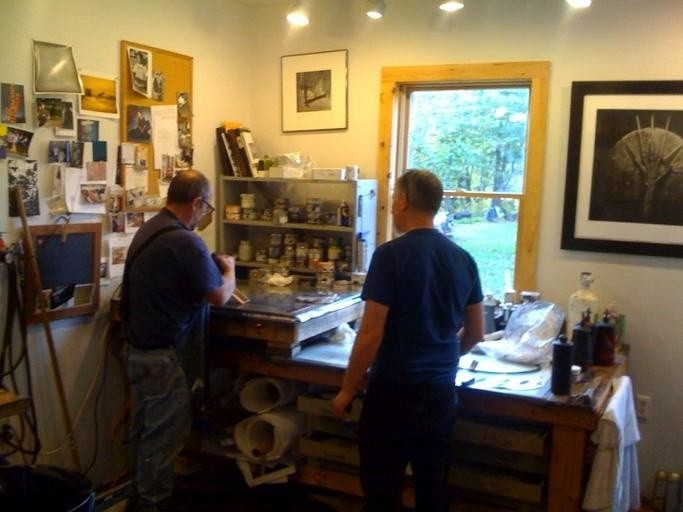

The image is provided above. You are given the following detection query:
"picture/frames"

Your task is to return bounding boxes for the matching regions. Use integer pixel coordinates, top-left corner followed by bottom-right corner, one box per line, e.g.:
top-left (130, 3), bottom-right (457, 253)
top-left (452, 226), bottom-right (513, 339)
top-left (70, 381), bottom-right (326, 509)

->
top-left (560, 81), bottom-right (682, 257)
top-left (283, 52), bottom-right (348, 133)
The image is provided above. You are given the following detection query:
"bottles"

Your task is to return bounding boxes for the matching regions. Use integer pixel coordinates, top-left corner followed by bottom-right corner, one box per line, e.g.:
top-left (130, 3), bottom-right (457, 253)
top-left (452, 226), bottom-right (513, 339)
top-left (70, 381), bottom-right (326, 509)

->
top-left (552, 308), bottom-right (616, 394)
top-left (224, 193), bottom-right (353, 274)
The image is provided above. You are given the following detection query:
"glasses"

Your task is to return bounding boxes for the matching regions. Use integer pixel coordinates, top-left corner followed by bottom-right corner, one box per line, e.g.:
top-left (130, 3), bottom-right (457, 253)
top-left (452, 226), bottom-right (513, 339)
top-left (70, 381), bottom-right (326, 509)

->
top-left (401, 169), bottom-right (412, 193)
top-left (202, 199), bottom-right (216, 216)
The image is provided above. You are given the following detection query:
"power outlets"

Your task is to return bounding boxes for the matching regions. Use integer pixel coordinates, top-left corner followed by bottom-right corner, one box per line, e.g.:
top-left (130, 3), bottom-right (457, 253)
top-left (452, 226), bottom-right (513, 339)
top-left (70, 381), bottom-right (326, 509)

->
top-left (0, 418), bottom-right (10, 440)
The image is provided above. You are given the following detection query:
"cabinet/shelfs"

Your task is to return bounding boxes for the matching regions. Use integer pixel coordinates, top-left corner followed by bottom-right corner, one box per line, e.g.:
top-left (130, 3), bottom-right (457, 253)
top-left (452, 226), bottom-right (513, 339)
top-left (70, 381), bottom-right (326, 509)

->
top-left (216, 176), bottom-right (378, 281)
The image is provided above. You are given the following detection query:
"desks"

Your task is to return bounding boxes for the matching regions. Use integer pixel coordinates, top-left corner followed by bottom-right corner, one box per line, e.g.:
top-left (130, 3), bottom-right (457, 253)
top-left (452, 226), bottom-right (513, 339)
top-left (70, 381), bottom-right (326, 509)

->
top-left (295, 309), bottom-right (634, 510)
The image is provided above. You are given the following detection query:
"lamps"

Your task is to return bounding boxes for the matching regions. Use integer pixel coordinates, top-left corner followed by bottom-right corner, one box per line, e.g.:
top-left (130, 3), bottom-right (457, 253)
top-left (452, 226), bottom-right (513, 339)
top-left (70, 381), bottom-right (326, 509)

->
top-left (286, 1), bottom-right (592, 27)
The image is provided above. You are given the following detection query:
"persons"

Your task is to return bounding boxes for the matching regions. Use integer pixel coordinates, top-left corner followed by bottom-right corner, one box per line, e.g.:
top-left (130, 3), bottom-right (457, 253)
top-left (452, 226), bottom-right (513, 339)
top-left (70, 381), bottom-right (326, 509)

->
top-left (119, 170), bottom-right (236, 512)
top-left (62, 104), bottom-right (73, 128)
top-left (129, 112), bottom-right (151, 139)
top-left (37, 104), bottom-right (49, 127)
top-left (133, 53), bottom-right (146, 92)
top-left (331, 169), bottom-right (484, 512)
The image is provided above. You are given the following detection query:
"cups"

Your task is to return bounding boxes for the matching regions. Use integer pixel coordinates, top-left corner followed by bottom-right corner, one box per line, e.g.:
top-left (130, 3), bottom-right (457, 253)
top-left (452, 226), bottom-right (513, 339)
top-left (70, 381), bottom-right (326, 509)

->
top-left (345, 165), bottom-right (359, 181)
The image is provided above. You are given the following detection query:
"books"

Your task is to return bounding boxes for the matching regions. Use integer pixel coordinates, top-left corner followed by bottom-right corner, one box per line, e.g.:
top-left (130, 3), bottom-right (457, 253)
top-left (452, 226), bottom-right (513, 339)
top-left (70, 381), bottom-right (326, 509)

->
top-left (217, 128), bottom-right (266, 177)
